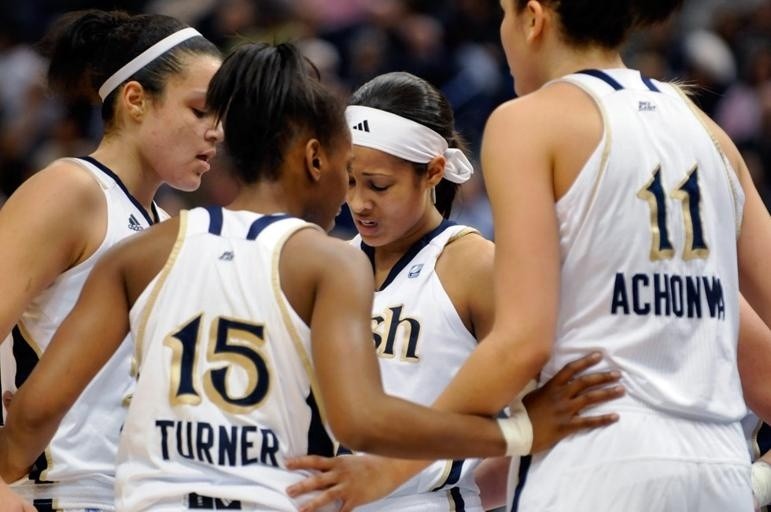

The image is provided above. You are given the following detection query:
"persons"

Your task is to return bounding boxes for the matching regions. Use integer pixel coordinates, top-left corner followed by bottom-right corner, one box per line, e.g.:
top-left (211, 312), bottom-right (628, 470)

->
top-left (2, 0), bottom-right (771, 512)
